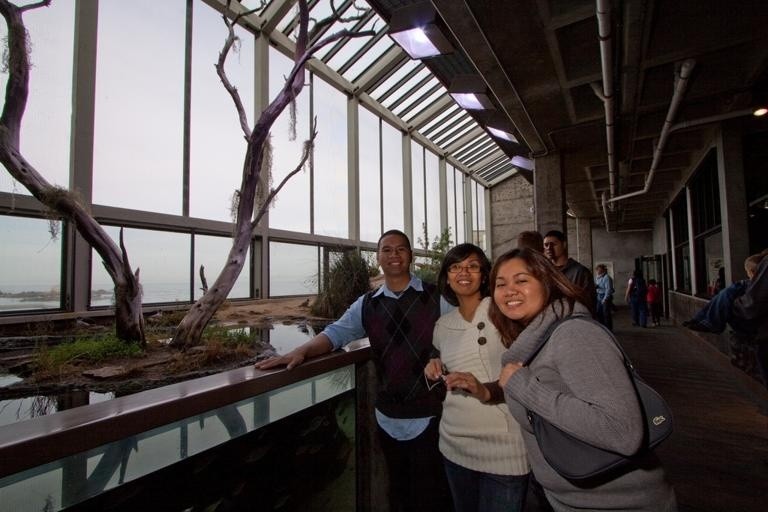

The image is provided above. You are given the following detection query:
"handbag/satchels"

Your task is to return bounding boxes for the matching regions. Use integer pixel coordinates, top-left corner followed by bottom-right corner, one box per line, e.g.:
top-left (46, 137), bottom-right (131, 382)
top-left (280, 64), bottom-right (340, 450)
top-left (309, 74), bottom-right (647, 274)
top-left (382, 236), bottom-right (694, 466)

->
top-left (520, 312), bottom-right (676, 482)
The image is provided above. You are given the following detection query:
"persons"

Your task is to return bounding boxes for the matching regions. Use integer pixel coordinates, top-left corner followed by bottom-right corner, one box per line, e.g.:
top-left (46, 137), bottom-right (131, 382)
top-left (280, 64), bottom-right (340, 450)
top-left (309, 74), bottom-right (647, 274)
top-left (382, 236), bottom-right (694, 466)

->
top-left (517, 230), bottom-right (544, 255)
top-left (645, 279), bottom-right (663, 327)
top-left (713, 267), bottom-right (726, 296)
top-left (253, 230), bottom-right (459, 511)
top-left (544, 229), bottom-right (597, 321)
top-left (421, 243), bottom-right (531, 512)
top-left (596, 263), bottom-right (616, 334)
top-left (486, 244), bottom-right (680, 512)
top-left (624, 268), bottom-right (648, 329)
top-left (682, 253), bottom-right (764, 336)
top-left (730, 256), bottom-right (768, 386)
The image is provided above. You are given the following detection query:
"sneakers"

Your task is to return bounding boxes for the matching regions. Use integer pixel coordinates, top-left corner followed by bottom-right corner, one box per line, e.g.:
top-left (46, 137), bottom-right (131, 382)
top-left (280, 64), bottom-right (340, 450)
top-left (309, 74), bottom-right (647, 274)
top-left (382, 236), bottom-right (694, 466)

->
top-left (681, 317), bottom-right (725, 336)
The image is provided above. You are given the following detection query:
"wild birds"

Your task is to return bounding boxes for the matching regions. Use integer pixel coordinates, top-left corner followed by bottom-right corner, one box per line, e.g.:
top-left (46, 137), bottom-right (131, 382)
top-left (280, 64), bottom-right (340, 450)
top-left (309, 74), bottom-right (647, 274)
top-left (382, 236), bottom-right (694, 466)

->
top-left (297, 298), bottom-right (310, 313)
top-left (149, 307), bottom-right (163, 320)
top-left (75, 315), bottom-right (96, 328)
top-left (298, 324), bottom-right (309, 335)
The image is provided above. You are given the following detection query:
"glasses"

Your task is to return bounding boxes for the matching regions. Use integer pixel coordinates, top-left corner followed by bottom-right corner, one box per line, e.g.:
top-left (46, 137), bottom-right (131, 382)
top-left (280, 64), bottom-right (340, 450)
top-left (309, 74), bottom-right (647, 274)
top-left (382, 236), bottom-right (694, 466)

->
top-left (446, 260), bottom-right (482, 275)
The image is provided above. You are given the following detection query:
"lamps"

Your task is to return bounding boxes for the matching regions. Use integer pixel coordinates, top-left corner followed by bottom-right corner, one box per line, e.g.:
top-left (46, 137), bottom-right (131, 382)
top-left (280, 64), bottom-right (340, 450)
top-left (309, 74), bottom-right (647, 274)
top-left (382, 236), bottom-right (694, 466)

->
top-left (447, 73), bottom-right (499, 110)
top-left (484, 109), bottom-right (524, 145)
top-left (387, 0), bottom-right (457, 60)
